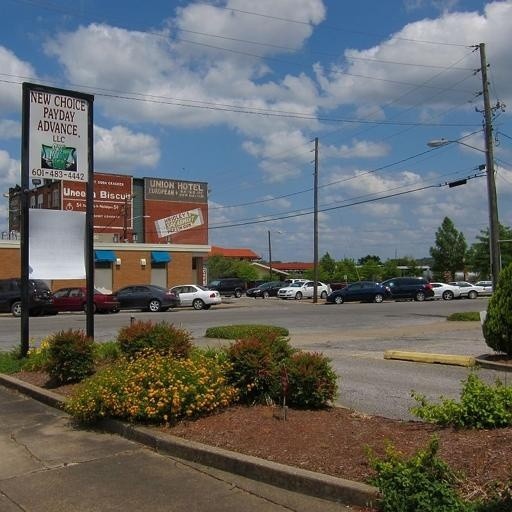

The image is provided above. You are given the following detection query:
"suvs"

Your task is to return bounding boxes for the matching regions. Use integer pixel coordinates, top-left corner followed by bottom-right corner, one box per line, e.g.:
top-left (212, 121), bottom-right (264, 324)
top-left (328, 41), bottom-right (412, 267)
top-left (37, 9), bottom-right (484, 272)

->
top-left (206, 278), bottom-right (244, 299)
top-left (380, 277), bottom-right (438, 302)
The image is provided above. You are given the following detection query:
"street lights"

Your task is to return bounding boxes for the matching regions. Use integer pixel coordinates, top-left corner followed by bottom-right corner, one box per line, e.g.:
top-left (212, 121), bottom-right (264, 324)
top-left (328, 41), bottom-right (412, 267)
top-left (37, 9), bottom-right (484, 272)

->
top-left (427, 44), bottom-right (502, 307)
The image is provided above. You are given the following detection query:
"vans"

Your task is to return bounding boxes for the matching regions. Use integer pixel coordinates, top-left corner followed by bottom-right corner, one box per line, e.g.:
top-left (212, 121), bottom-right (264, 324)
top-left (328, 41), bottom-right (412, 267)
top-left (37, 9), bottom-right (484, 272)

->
top-left (0, 277), bottom-right (58, 318)
top-left (284, 278), bottom-right (308, 284)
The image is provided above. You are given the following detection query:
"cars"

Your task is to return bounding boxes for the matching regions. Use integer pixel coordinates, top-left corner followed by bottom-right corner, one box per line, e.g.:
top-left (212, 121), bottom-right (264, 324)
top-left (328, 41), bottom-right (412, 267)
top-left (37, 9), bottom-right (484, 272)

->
top-left (166, 284), bottom-right (221, 310)
top-left (278, 280), bottom-right (332, 300)
top-left (246, 280), bottom-right (286, 299)
top-left (111, 284), bottom-right (181, 313)
top-left (51, 287), bottom-right (122, 314)
top-left (324, 280), bottom-right (492, 305)
